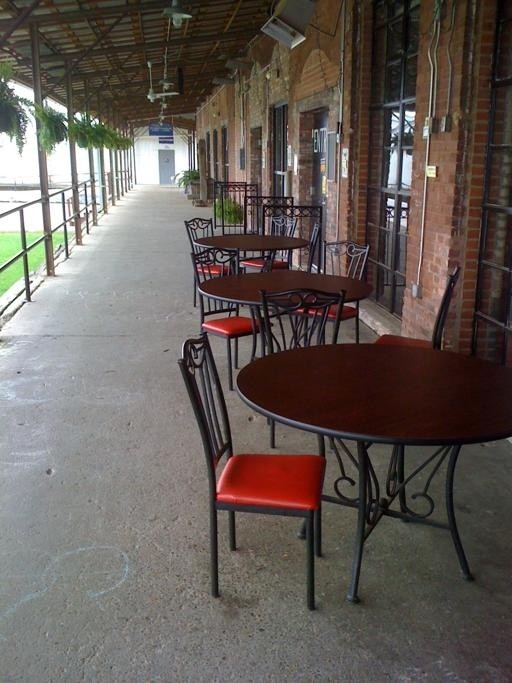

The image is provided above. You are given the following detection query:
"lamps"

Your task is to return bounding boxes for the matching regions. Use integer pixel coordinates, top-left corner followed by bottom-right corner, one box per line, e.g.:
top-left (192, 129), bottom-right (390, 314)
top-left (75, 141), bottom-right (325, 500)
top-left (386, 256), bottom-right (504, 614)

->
top-left (158, 0), bottom-right (193, 126)
top-left (260, 0), bottom-right (315, 49)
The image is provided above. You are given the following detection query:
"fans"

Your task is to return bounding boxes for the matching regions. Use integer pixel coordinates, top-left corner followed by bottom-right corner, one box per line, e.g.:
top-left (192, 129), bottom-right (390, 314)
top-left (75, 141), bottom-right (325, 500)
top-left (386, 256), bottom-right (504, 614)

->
top-left (146, 61), bottom-right (180, 102)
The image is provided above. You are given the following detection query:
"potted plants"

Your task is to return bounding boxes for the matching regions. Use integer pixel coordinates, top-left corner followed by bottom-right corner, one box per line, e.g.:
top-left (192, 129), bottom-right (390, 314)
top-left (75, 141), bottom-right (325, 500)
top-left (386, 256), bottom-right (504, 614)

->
top-left (0, 59), bottom-right (133, 156)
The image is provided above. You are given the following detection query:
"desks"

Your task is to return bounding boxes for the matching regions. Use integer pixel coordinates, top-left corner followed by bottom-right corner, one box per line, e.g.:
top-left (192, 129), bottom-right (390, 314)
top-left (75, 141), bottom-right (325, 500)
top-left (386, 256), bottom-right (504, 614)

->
top-left (235, 343), bottom-right (512, 602)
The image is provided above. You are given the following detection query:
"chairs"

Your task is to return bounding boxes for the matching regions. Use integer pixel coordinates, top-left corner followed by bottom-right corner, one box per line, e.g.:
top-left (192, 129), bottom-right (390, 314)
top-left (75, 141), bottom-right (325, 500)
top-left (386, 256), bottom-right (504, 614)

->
top-left (178, 332), bottom-right (327, 610)
top-left (175, 215), bottom-right (373, 391)
top-left (373, 264), bottom-right (461, 349)
